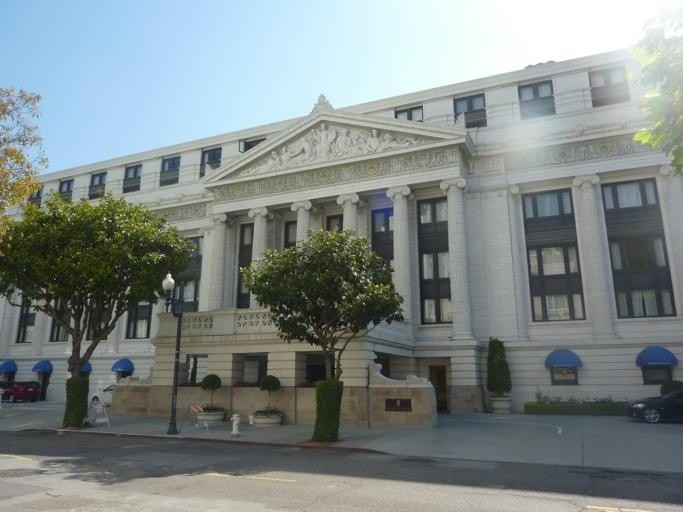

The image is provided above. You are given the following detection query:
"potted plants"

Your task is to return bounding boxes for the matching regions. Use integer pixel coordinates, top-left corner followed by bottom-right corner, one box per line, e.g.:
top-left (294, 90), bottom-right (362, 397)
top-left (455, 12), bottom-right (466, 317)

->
top-left (253, 375), bottom-right (284, 426)
top-left (195, 374), bottom-right (227, 425)
top-left (486, 336), bottom-right (513, 415)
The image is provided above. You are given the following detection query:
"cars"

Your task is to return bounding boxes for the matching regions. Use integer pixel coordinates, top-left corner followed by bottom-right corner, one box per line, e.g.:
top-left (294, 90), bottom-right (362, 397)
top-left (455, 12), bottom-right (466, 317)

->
top-left (625, 387), bottom-right (683, 424)
top-left (0, 382), bottom-right (41, 402)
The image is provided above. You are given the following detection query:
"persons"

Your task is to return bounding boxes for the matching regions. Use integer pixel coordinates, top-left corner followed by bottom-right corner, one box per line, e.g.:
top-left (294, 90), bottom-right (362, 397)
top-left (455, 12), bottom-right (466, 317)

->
top-left (86, 395), bottom-right (105, 430)
top-left (237, 122), bottom-right (424, 179)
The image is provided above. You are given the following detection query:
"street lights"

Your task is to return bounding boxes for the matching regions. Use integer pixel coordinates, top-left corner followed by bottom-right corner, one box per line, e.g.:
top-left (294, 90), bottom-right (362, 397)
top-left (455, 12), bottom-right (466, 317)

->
top-left (162, 272), bottom-right (184, 434)
